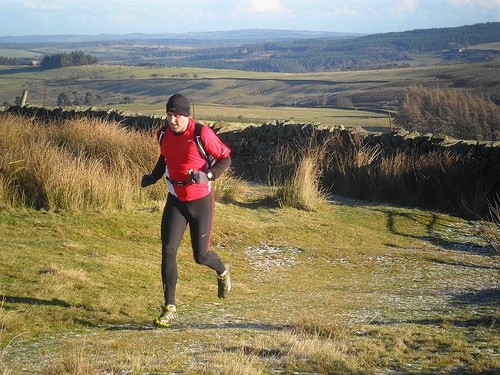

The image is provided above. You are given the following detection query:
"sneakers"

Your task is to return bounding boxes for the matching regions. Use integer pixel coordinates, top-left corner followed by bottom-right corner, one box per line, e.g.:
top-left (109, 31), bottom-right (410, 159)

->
top-left (153, 304), bottom-right (181, 327)
top-left (216, 263), bottom-right (231, 300)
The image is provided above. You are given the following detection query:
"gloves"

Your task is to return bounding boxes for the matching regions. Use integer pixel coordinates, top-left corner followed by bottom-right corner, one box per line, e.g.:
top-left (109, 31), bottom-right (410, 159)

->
top-left (191, 170), bottom-right (208, 182)
top-left (141, 174), bottom-right (154, 187)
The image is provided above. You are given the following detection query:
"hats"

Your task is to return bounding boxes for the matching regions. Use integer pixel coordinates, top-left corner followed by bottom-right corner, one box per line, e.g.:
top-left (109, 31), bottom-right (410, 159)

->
top-left (166, 93), bottom-right (191, 118)
